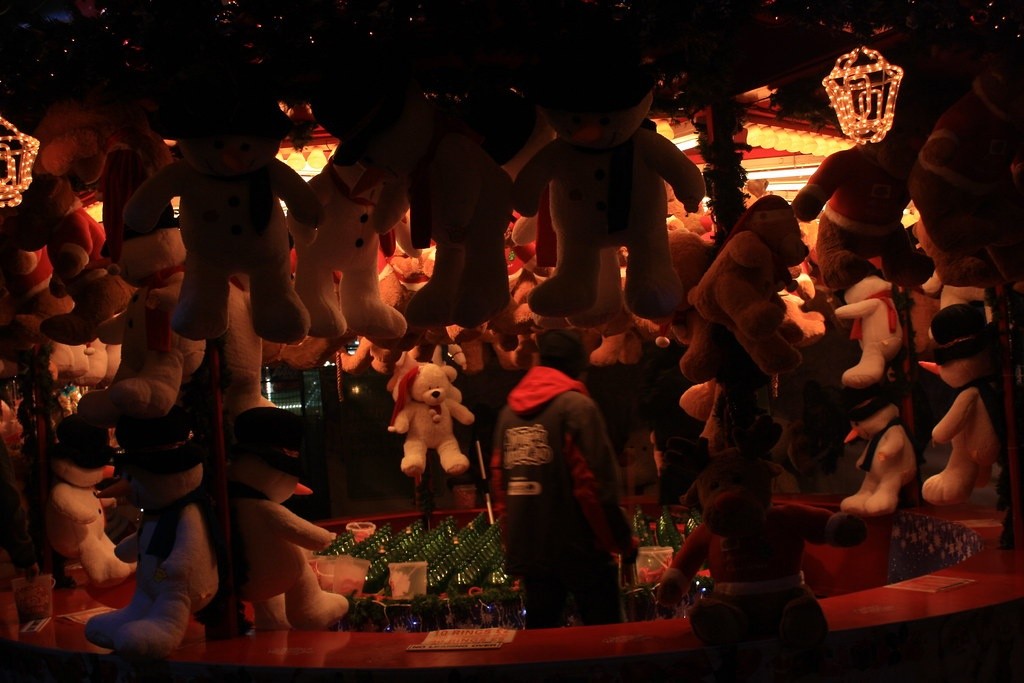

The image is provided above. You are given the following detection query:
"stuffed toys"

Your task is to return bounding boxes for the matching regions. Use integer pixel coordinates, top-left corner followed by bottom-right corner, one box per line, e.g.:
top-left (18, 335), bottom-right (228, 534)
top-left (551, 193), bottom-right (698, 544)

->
top-left (1, 0), bottom-right (1024, 683)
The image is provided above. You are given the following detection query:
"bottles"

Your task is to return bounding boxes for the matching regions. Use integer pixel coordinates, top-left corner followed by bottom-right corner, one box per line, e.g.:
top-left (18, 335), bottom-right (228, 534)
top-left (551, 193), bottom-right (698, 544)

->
top-left (633, 507), bottom-right (701, 556)
top-left (318, 514), bottom-right (527, 632)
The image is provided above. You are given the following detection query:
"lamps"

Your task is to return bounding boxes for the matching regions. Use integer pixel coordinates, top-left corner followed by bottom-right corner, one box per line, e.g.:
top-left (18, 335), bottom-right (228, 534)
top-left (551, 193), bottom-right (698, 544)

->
top-left (821, 45), bottom-right (904, 147)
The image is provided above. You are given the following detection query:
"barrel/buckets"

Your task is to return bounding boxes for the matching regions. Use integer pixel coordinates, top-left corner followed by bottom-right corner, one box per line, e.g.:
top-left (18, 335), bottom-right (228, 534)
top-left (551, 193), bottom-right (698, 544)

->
top-left (454, 484), bottom-right (475, 508)
top-left (635, 546), bottom-right (674, 585)
